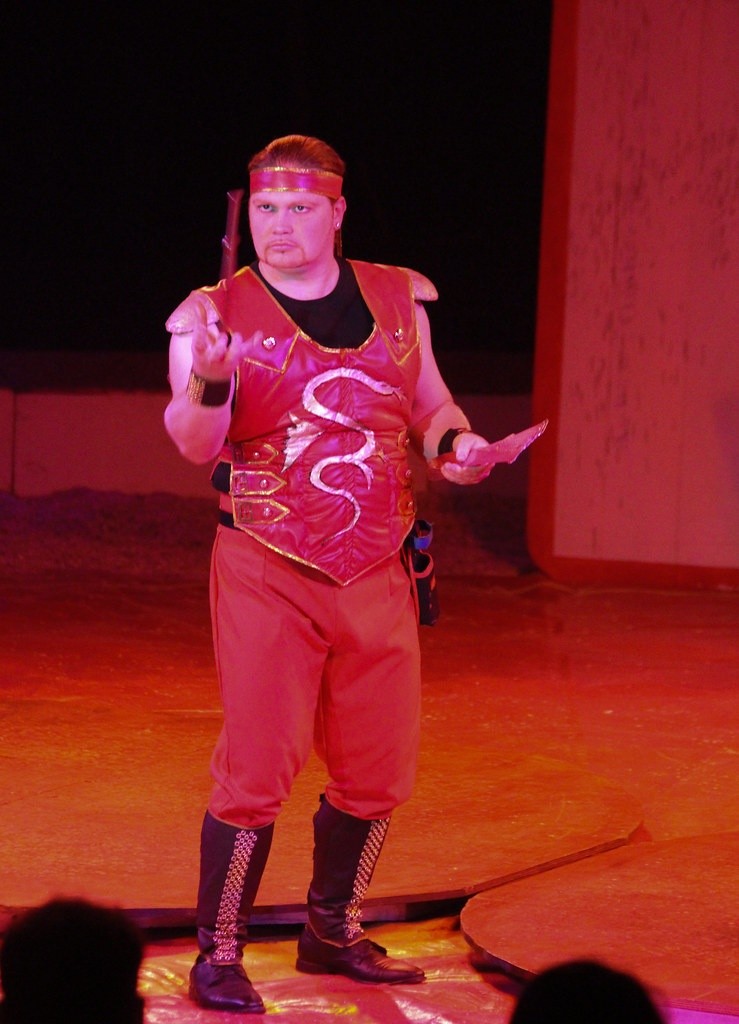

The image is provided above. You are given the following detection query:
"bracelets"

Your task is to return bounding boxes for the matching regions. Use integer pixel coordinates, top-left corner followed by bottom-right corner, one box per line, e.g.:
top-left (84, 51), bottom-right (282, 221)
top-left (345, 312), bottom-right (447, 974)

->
top-left (184, 370), bottom-right (231, 408)
top-left (437, 426), bottom-right (473, 456)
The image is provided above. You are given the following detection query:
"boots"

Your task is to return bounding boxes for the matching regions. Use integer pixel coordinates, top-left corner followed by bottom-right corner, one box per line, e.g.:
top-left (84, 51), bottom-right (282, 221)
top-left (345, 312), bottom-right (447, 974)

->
top-left (188, 810), bottom-right (274, 1014)
top-left (295, 793), bottom-right (426, 983)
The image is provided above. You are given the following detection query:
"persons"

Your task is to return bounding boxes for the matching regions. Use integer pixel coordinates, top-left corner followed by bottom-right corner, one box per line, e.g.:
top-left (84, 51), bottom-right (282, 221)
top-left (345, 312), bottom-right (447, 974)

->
top-left (0, 899), bottom-right (148, 1024)
top-left (164, 134), bottom-right (496, 1015)
top-left (509, 960), bottom-right (664, 1024)
